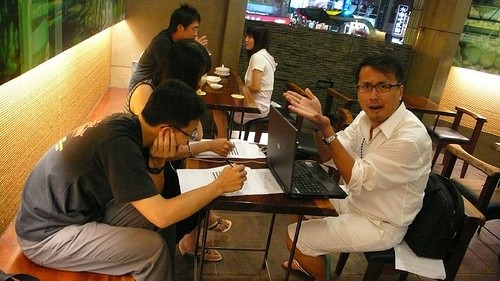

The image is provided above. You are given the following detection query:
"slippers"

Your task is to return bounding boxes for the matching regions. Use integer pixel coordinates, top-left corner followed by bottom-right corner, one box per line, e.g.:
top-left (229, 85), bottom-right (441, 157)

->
top-left (281, 259), bottom-right (310, 276)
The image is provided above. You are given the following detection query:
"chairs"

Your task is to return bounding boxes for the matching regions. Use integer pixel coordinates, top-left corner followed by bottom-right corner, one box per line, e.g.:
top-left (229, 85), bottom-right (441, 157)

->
top-left (323, 86), bottom-right (356, 132)
top-left (426, 105), bottom-right (487, 178)
top-left (335, 175), bottom-right (482, 281)
top-left (440, 144), bottom-right (500, 233)
top-left (244, 81), bottom-right (309, 143)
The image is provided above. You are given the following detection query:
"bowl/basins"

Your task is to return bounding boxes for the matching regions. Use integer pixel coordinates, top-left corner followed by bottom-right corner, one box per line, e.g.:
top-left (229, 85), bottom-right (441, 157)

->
top-left (207, 76), bottom-right (221, 85)
top-left (215, 67), bottom-right (229, 74)
top-left (210, 83), bottom-right (223, 89)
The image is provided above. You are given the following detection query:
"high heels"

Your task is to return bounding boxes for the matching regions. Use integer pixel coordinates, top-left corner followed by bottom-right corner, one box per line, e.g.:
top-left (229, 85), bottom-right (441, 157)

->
top-left (206, 215), bottom-right (231, 232)
top-left (179, 243), bottom-right (222, 261)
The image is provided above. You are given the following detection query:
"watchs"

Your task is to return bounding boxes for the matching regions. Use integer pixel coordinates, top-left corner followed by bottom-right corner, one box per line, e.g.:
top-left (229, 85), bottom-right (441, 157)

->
top-left (148, 167), bottom-right (163, 174)
top-left (322, 134), bottom-right (337, 145)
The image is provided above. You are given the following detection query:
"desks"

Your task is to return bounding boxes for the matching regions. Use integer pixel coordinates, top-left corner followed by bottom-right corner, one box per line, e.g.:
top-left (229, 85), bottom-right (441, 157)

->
top-left (196, 66), bottom-right (261, 137)
top-left (184, 137), bottom-right (339, 281)
top-left (401, 94), bottom-right (457, 125)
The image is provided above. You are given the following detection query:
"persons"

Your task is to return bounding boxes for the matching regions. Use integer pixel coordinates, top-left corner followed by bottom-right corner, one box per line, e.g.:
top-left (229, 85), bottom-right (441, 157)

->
top-left (129, 2), bottom-right (207, 88)
top-left (282, 54), bottom-right (432, 281)
top-left (214, 23), bottom-right (276, 139)
top-left (122, 38), bottom-right (234, 261)
top-left (15, 79), bottom-right (248, 281)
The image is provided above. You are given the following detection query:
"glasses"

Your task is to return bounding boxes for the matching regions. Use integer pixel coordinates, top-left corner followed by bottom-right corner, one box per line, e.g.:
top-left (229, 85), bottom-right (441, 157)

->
top-left (160, 122), bottom-right (198, 140)
top-left (356, 81), bottom-right (403, 93)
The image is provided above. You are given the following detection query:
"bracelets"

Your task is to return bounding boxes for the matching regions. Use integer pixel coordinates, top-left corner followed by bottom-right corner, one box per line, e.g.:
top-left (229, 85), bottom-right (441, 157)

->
top-left (188, 145), bottom-right (192, 158)
top-left (312, 126), bottom-right (319, 131)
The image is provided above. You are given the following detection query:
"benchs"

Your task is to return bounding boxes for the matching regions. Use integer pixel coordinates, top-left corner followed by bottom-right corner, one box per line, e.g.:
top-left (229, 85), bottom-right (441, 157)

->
top-left (0, 87), bottom-right (136, 281)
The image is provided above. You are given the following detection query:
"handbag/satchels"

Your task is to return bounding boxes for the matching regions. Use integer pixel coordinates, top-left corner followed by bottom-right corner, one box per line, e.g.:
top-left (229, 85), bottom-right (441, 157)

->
top-left (403, 173), bottom-right (465, 261)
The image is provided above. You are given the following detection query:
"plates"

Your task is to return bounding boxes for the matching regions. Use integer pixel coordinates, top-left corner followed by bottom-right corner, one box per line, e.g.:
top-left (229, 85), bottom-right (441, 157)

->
top-left (214, 71), bottom-right (230, 77)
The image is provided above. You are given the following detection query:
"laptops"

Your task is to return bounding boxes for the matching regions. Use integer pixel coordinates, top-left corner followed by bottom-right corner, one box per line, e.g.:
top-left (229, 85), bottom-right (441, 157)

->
top-left (266, 105), bottom-right (348, 199)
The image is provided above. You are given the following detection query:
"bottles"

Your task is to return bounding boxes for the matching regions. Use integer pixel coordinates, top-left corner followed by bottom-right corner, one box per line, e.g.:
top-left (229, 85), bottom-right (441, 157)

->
top-left (292, 16), bottom-right (367, 36)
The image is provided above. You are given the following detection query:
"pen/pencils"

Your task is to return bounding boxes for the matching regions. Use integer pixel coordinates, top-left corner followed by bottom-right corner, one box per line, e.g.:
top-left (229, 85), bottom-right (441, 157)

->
top-left (226, 136), bottom-right (229, 141)
top-left (225, 159), bottom-right (233, 167)
